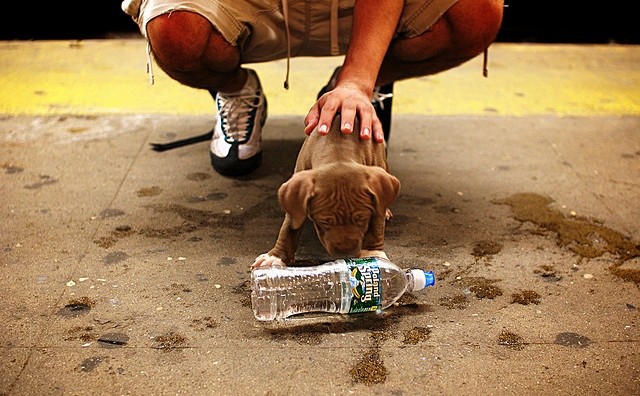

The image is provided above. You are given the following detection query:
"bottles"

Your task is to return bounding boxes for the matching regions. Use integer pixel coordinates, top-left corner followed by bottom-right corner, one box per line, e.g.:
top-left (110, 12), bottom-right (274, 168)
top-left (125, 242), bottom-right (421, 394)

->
top-left (251, 257), bottom-right (434, 321)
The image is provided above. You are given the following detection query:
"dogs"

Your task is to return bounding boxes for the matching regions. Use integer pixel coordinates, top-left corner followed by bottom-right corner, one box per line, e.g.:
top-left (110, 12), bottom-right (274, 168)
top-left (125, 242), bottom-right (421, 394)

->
top-left (249, 113), bottom-right (402, 273)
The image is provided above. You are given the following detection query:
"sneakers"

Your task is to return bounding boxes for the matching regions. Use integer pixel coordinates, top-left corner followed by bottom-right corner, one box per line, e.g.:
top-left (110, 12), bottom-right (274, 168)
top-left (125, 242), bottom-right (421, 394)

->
top-left (210, 68), bottom-right (267, 175)
top-left (317, 66), bottom-right (393, 141)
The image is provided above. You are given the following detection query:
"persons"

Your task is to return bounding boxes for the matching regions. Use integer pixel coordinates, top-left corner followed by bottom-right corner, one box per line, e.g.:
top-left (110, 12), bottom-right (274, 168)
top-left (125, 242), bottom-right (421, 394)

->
top-left (121, 0), bottom-right (505, 179)
